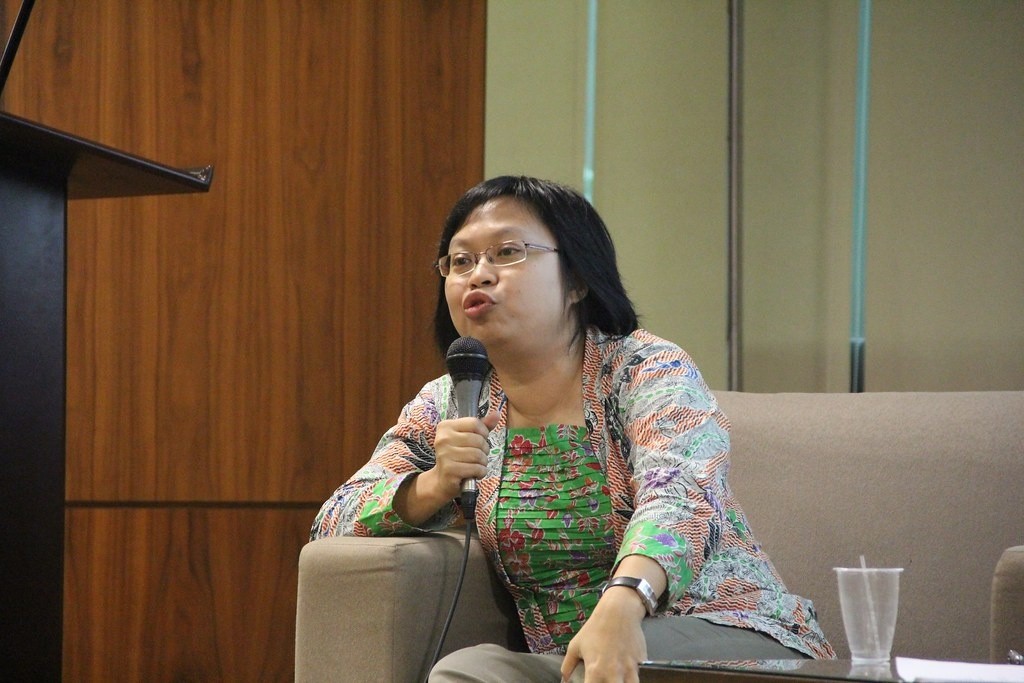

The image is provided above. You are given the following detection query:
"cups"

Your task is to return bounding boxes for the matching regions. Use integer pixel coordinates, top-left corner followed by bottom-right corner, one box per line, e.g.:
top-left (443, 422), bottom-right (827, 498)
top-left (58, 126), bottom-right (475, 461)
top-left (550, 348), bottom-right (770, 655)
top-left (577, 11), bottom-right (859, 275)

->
top-left (832, 567), bottom-right (903, 662)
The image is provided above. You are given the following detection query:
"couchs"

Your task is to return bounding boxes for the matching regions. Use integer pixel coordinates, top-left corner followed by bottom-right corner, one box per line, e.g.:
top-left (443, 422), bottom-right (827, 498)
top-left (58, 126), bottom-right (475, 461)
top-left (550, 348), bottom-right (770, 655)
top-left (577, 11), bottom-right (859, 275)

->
top-left (294, 390), bottom-right (1023, 683)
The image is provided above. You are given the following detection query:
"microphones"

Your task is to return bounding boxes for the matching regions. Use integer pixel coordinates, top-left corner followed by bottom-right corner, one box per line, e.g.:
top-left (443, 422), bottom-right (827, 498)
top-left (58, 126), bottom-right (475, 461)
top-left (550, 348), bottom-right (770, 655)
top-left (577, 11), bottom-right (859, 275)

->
top-left (445, 336), bottom-right (490, 520)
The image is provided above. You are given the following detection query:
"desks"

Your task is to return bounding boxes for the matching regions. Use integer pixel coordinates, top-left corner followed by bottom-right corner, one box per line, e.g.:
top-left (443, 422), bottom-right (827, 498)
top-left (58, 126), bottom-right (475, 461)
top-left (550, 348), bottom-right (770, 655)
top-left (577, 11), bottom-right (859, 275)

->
top-left (636, 658), bottom-right (1023, 683)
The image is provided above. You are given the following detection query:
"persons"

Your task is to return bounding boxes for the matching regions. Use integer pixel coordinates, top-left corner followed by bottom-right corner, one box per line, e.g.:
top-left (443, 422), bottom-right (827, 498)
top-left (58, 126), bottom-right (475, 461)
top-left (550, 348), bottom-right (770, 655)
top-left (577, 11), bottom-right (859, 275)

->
top-left (309, 175), bottom-right (839, 683)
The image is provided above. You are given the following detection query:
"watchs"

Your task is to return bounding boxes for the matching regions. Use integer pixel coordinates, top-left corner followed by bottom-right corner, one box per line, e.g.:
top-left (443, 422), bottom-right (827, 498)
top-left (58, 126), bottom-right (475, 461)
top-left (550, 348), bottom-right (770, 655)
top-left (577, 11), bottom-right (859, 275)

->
top-left (601, 576), bottom-right (659, 617)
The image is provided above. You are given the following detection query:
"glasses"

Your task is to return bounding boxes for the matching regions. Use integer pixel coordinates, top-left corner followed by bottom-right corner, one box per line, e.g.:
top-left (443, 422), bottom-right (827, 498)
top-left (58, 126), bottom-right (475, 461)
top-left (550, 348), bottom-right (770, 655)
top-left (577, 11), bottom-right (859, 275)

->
top-left (436, 240), bottom-right (559, 278)
